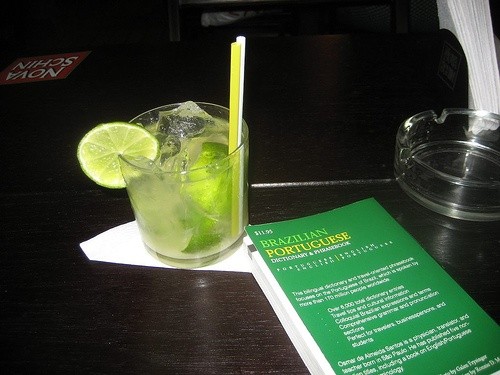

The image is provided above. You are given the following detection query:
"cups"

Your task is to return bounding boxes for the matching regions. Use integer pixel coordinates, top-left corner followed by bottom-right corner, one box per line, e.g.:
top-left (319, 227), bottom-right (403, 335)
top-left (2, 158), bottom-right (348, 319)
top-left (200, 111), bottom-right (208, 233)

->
top-left (119, 101), bottom-right (249, 271)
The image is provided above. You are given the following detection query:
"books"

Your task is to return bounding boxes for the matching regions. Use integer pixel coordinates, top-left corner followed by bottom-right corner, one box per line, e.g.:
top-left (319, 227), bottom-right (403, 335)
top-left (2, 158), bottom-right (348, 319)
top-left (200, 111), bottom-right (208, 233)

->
top-left (242, 196), bottom-right (500, 375)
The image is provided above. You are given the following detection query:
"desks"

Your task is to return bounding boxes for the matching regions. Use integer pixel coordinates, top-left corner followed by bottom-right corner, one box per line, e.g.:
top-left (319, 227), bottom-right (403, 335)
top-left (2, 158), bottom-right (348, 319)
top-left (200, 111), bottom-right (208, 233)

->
top-left (1, 34), bottom-right (499, 373)
